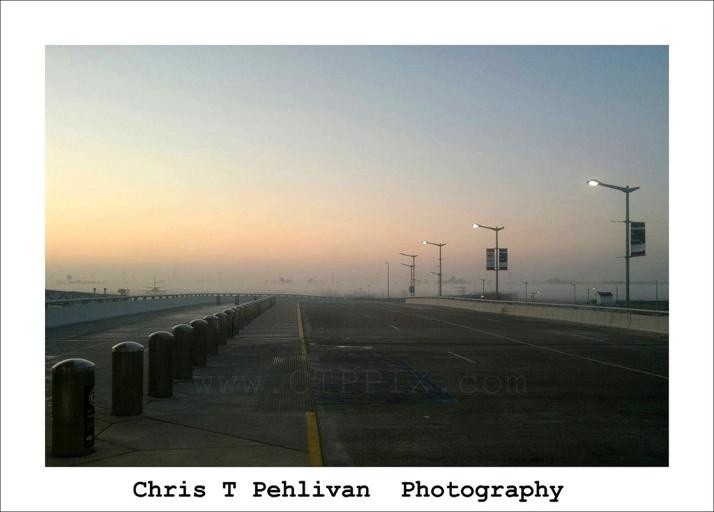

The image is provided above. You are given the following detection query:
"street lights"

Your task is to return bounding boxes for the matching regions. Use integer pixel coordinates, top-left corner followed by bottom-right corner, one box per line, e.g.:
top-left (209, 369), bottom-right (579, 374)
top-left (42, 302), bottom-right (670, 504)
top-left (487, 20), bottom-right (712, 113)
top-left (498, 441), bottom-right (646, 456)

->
top-left (424, 241), bottom-right (446, 297)
top-left (384, 262), bottom-right (390, 300)
top-left (586, 179), bottom-right (639, 306)
top-left (399, 252), bottom-right (417, 298)
top-left (473, 223), bottom-right (504, 299)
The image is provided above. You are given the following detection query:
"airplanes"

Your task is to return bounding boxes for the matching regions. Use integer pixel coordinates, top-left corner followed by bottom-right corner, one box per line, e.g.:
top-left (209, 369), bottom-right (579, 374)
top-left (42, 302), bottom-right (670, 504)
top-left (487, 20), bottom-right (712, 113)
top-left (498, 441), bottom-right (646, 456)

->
top-left (130, 278), bottom-right (183, 294)
